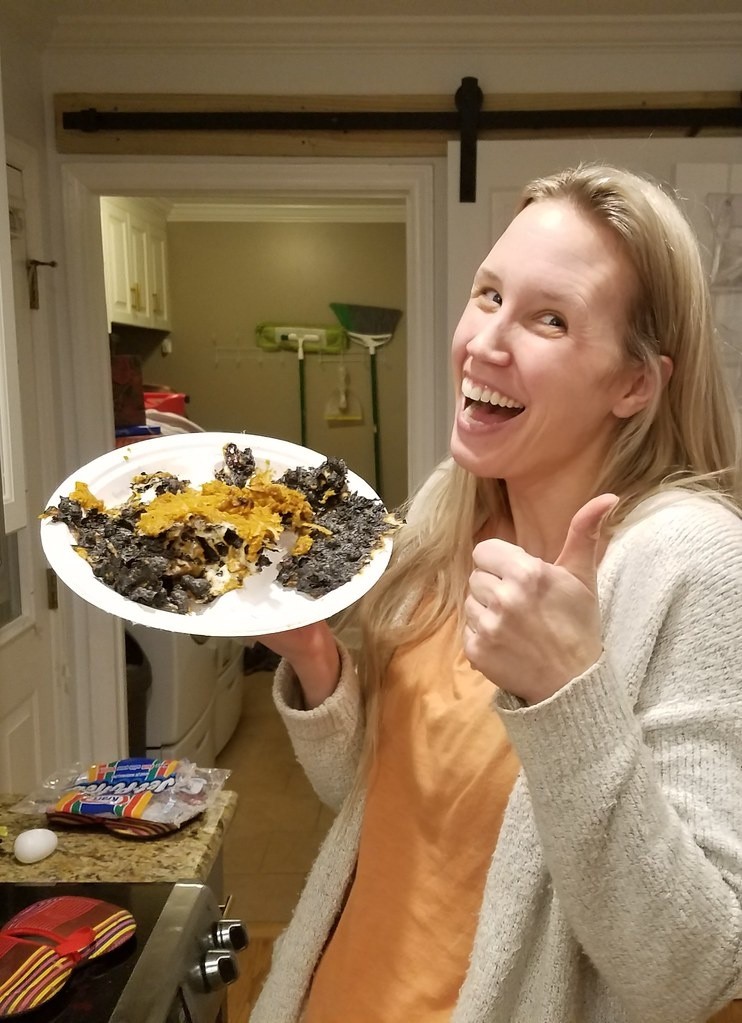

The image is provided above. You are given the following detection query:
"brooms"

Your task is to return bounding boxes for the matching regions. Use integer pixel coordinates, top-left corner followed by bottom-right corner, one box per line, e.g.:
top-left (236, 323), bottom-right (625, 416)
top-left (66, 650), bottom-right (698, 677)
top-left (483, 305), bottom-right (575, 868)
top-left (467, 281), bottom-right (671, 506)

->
top-left (327, 298), bottom-right (402, 511)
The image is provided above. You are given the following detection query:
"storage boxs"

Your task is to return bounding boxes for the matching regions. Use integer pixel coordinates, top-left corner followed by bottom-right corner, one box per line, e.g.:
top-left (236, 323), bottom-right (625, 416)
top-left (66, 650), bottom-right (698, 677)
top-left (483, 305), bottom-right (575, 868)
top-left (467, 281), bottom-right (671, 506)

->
top-left (111, 354), bottom-right (146, 429)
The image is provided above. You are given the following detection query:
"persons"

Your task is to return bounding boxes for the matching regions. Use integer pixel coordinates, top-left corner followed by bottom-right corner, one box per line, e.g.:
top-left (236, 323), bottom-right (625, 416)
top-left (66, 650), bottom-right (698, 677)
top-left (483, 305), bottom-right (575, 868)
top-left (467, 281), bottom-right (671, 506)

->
top-left (254, 157), bottom-right (740, 1023)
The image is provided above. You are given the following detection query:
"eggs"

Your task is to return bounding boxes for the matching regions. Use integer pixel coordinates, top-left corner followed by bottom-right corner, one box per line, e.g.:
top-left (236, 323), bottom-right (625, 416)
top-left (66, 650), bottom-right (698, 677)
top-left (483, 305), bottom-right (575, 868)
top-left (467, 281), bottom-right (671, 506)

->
top-left (14, 829), bottom-right (58, 864)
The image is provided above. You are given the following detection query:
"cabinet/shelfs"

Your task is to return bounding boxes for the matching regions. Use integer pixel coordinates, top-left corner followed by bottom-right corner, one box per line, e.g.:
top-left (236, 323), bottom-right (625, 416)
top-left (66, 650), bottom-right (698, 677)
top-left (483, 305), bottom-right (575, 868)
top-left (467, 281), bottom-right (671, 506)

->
top-left (99, 195), bottom-right (175, 333)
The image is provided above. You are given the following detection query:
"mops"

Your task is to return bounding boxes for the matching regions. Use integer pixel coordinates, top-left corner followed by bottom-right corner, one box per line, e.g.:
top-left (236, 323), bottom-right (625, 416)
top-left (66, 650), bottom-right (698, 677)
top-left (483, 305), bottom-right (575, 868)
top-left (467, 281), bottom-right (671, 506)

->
top-left (254, 318), bottom-right (351, 454)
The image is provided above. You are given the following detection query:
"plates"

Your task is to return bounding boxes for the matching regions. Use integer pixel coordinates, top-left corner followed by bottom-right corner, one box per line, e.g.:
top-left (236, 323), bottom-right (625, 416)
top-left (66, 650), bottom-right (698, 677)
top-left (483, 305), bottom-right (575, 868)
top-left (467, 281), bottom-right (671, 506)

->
top-left (42, 432), bottom-right (398, 638)
